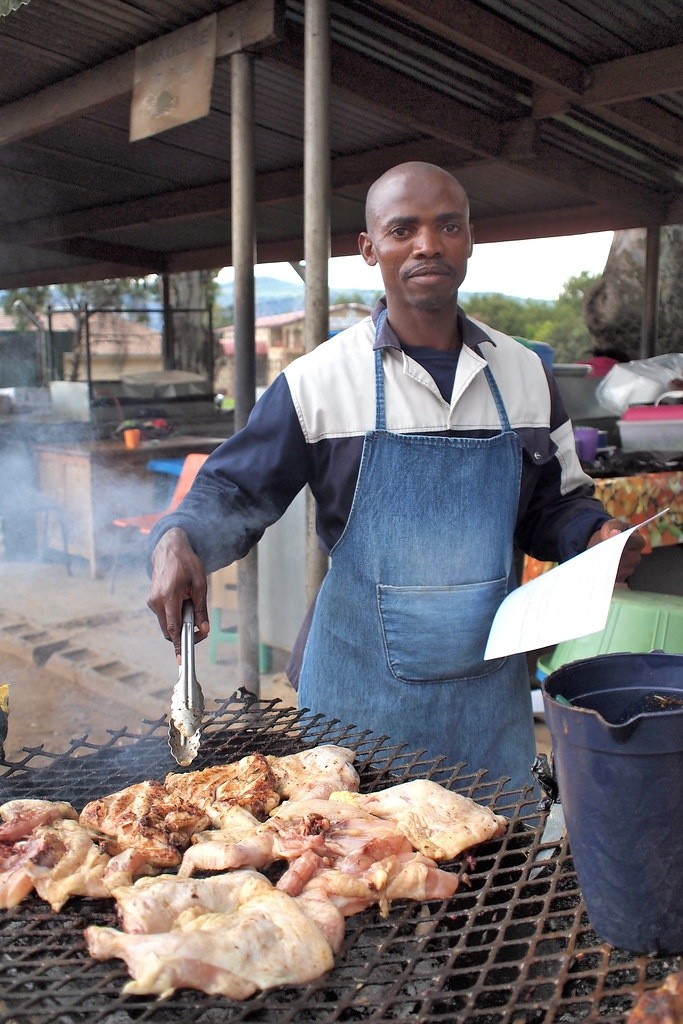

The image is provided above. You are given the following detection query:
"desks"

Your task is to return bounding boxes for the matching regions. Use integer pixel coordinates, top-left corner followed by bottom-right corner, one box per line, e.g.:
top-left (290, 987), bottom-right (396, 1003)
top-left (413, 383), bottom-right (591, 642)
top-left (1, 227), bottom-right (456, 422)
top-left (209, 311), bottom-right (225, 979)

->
top-left (145, 459), bottom-right (182, 505)
top-left (521, 470), bottom-right (683, 588)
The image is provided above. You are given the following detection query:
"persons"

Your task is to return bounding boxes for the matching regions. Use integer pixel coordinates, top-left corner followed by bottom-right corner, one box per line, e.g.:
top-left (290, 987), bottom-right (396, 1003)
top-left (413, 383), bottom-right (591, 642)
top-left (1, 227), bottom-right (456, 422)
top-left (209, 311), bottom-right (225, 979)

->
top-left (148, 160), bottom-right (646, 829)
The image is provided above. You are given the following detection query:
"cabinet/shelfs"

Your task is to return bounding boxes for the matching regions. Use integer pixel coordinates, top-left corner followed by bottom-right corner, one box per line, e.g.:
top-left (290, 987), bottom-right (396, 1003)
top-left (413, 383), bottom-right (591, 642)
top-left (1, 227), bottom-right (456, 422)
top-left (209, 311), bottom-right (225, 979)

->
top-left (38, 435), bottom-right (226, 578)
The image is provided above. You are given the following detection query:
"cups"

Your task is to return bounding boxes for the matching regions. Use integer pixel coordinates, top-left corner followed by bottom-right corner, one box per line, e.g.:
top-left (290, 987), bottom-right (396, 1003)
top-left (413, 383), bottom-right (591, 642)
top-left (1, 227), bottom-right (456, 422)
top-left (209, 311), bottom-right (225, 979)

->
top-left (572, 427), bottom-right (598, 465)
top-left (123, 429), bottom-right (140, 448)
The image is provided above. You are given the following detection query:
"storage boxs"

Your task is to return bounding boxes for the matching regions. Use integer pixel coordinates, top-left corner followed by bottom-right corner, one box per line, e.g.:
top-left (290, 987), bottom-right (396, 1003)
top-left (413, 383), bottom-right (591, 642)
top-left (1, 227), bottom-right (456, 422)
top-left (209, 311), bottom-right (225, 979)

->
top-left (616, 404), bottom-right (683, 456)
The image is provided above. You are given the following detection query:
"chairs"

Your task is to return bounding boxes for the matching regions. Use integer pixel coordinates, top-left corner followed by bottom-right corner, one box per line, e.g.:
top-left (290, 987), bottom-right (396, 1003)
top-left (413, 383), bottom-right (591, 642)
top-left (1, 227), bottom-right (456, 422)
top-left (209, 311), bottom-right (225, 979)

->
top-left (107, 453), bottom-right (209, 585)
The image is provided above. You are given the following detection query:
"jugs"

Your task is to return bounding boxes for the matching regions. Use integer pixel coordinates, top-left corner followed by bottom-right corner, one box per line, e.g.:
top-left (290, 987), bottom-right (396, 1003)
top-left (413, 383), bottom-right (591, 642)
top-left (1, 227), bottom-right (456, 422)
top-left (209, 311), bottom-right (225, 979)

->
top-left (542, 650), bottom-right (683, 953)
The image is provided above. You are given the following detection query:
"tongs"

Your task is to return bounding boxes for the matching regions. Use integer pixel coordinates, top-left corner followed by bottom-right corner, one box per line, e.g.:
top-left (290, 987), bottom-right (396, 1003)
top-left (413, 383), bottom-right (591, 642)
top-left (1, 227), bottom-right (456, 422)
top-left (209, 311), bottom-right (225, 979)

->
top-left (166, 610), bottom-right (204, 764)
